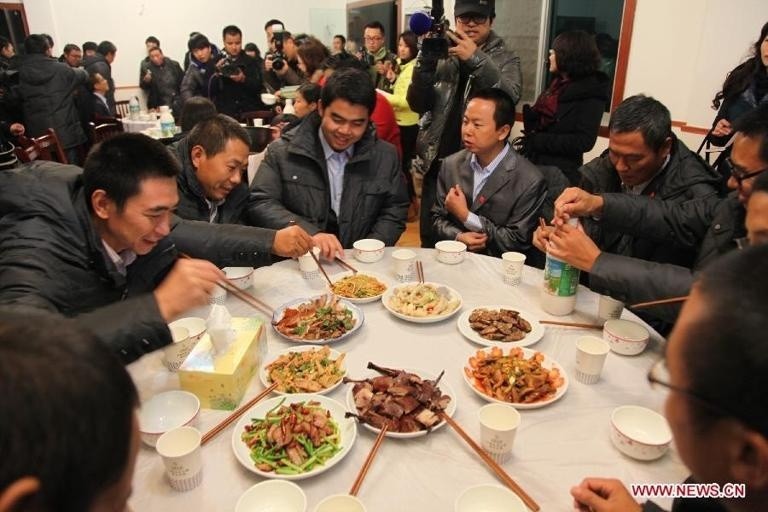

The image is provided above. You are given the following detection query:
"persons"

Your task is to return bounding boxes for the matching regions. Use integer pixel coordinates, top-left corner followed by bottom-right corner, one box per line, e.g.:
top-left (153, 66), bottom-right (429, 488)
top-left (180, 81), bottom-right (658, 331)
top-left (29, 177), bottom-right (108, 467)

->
top-left (0, 0), bottom-right (768, 512)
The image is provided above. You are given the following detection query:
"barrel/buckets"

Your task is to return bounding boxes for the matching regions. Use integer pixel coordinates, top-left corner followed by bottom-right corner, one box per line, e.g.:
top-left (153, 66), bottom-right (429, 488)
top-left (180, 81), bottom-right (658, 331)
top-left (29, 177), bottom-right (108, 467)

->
top-left (542, 212), bottom-right (579, 313)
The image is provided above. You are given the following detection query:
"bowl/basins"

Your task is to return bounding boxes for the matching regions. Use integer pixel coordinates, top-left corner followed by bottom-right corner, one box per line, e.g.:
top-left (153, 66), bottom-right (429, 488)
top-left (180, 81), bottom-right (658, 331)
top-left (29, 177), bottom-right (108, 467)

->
top-left (157, 317), bottom-right (206, 344)
top-left (610, 405), bottom-right (673, 463)
top-left (432, 239), bottom-right (466, 265)
top-left (354, 238), bottom-right (384, 264)
top-left (221, 264), bottom-right (256, 288)
top-left (134, 391), bottom-right (201, 446)
top-left (603, 319), bottom-right (652, 358)
top-left (260, 94), bottom-right (276, 107)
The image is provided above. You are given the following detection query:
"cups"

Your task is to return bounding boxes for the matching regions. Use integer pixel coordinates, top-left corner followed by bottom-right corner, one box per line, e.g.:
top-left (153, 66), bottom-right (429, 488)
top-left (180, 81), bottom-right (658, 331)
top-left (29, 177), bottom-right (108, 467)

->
top-left (476, 401), bottom-right (523, 466)
top-left (575, 334), bottom-right (613, 388)
top-left (234, 480), bottom-right (366, 511)
top-left (153, 426), bottom-right (203, 491)
top-left (595, 288), bottom-right (625, 327)
top-left (164, 328), bottom-right (188, 375)
top-left (500, 250), bottom-right (526, 283)
top-left (239, 119), bottom-right (269, 129)
top-left (453, 483), bottom-right (527, 511)
top-left (392, 248), bottom-right (415, 283)
top-left (298, 244), bottom-right (322, 279)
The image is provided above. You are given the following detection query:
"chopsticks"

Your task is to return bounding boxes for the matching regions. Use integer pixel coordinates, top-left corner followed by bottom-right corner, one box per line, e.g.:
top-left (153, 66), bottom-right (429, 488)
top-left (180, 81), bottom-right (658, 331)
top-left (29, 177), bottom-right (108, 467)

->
top-left (335, 246), bottom-right (360, 274)
top-left (539, 321), bottom-right (605, 330)
top-left (417, 260), bottom-right (425, 284)
top-left (434, 406), bottom-right (545, 511)
top-left (173, 247), bottom-right (280, 318)
top-left (346, 424), bottom-right (389, 496)
top-left (200, 382), bottom-right (277, 446)
top-left (289, 220), bottom-right (339, 289)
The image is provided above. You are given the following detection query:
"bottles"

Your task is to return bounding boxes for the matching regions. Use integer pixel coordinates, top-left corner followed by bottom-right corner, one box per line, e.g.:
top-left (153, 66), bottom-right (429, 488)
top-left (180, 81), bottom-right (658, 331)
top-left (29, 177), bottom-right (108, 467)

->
top-left (544, 219), bottom-right (581, 316)
top-left (283, 98), bottom-right (298, 116)
top-left (129, 93), bottom-right (178, 137)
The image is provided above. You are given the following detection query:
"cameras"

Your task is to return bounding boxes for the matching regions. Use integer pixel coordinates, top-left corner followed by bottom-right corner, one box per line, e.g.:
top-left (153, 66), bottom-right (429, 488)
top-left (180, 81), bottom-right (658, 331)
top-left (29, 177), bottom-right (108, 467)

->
top-left (221, 54), bottom-right (238, 77)
top-left (272, 24), bottom-right (287, 70)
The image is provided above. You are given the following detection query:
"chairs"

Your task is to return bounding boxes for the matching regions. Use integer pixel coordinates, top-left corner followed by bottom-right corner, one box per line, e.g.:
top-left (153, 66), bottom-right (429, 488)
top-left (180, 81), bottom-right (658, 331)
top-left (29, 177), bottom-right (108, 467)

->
top-left (15, 97), bottom-right (139, 165)
top-left (526, 163), bottom-right (567, 236)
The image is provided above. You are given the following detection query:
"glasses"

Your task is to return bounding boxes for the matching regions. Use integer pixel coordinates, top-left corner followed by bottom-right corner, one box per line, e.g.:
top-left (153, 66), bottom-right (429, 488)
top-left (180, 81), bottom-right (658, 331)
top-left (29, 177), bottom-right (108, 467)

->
top-left (726, 157), bottom-right (768, 184)
top-left (647, 357), bottom-right (717, 407)
top-left (457, 15), bottom-right (488, 24)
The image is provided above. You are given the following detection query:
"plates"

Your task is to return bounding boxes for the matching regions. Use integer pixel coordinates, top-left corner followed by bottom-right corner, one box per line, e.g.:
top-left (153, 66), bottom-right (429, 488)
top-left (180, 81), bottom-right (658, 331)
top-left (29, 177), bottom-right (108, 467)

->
top-left (382, 280), bottom-right (462, 327)
top-left (327, 273), bottom-right (389, 305)
top-left (456, 302), bottom-right (545, 349)
top-left (272, 295), bottom-right (368, 344)
top-left (462, 346), bottom-right (567, 412)
top-left (258, 347), bottom-right (350, 396)
top-left (343, 375), bottom-right (458, 437)
top-left (232, 394), bottom-right (357, 480)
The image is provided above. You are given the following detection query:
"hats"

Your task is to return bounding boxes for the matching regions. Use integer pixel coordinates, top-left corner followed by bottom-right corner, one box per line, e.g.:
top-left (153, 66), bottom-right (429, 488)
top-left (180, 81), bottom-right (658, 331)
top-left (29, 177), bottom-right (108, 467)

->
top-left (454, 0), bottom-right (495, 17)
